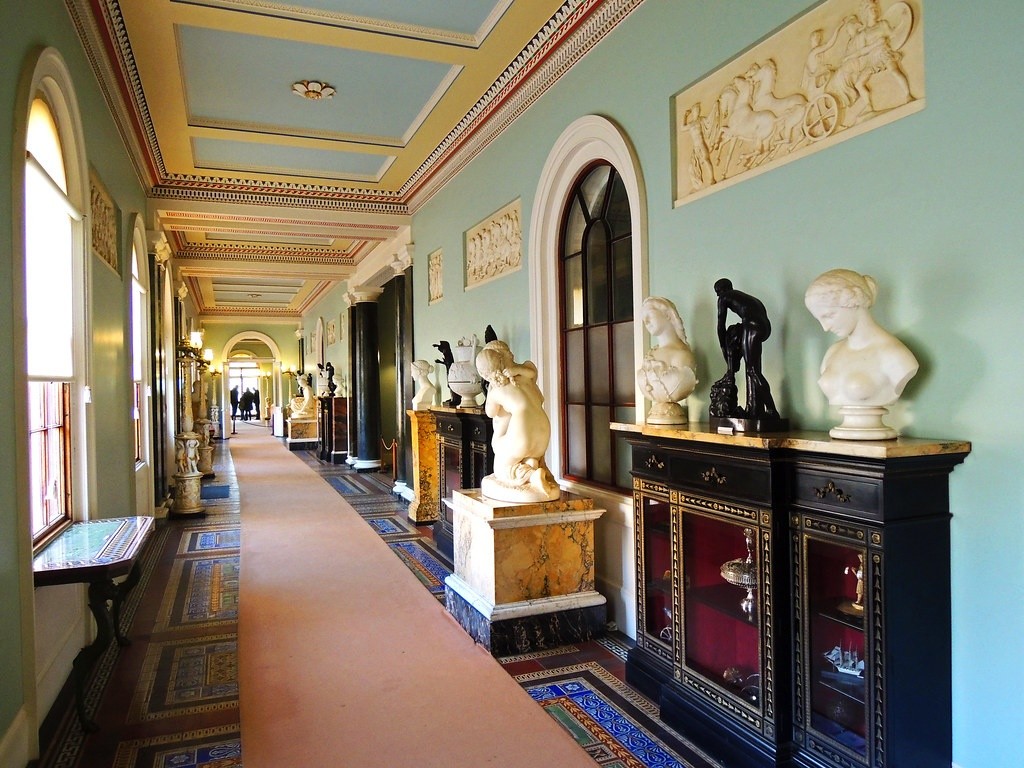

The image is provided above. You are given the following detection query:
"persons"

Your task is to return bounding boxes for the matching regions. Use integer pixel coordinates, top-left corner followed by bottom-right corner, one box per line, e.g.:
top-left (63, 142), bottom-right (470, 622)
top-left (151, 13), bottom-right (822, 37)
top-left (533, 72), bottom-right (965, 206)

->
top-left (476, 341), bottom-right (559, 498)
top-left (239, 387), bottom-right (260, 421)
top-left (804, 0), bottom-right (917, 118)
top-left (176, 439), bottom-right (202, 473)
top-left (714, 278), bottom-right (780, 418)
top-left (296, 361), bottom-right (346, 415)
top-left (682, 103), bottom-right (717, 194)
top-left (231, 385), bottom-right (239, 420)
top-left (804, 268), bottom-right (919, 407)
top-left (638, 296), bottom-right (698, 404)
top-left (192, 381), bottom-right (208, 402)
top-left (411, 360), bottom-right (437, 403)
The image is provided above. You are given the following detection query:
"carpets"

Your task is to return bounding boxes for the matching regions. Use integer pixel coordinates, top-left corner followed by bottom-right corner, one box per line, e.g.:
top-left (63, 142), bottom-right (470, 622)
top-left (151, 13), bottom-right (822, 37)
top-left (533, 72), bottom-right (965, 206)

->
top-left (200, 485), bottom-right (229, 499)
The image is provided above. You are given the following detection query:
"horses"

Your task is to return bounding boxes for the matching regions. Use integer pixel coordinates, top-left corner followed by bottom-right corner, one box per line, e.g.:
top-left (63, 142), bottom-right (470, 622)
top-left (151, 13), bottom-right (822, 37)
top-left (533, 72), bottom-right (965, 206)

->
top-left (708, 58), bottom-right (808, 181)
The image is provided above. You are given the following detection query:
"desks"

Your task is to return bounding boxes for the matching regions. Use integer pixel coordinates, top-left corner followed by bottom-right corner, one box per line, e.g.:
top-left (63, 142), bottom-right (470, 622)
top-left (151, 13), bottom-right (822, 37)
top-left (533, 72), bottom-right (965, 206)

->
top-left (27, 516), bottom-right (155, 736)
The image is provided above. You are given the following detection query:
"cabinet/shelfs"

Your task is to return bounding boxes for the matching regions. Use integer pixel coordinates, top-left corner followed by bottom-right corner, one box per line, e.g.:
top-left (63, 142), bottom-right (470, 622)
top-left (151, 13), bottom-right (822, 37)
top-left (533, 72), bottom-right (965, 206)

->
top-left (319, 396), bottom-right (354, 464)
top-left (608, 420), bottom-right (974, 768)
top-left (428, 409), bottom-right (496, 560)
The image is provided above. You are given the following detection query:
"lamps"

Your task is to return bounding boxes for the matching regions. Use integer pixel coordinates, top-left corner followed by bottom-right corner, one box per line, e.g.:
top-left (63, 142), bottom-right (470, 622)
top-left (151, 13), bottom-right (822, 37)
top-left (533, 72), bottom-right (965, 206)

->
top-left (248, 294), bottom-right (262, 300)
top-left (291, 80), bottom-right (337, 100)
top-left (177, 331), bottom-right (223, 380)
top-left (258, 364), bottom-right (300, 381)
